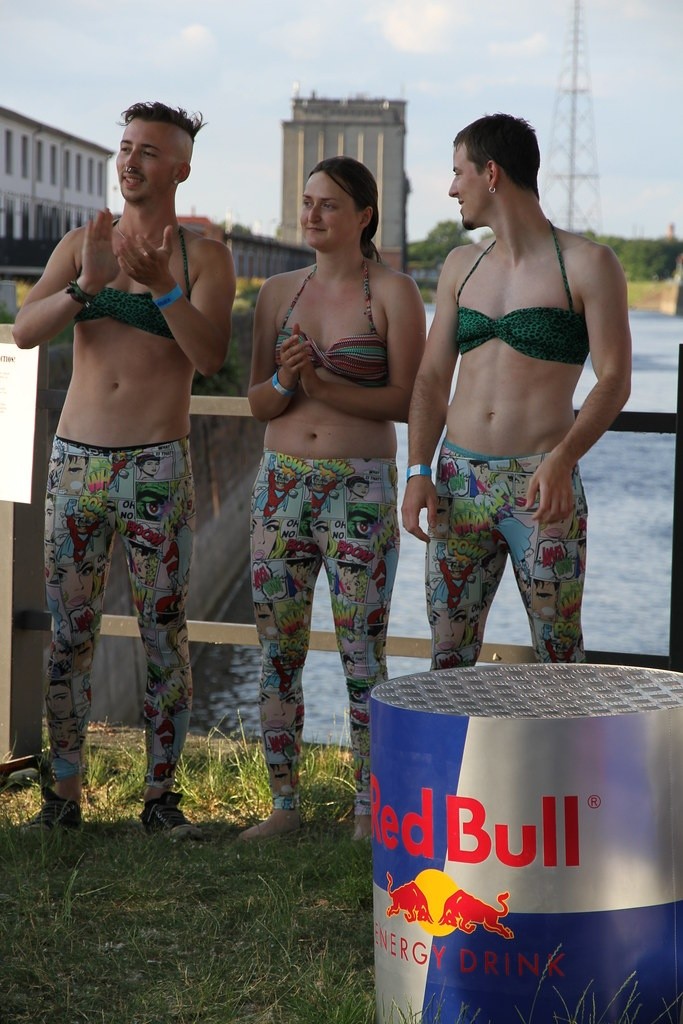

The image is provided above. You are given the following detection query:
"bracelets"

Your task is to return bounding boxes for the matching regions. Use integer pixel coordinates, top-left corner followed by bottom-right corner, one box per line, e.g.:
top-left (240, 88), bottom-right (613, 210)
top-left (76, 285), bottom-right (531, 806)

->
top-left (154, 284), bottom-right (183, 309)
top-left (405, 463), bottom-right (431, 482)
top-left (272, 371), bottom-right (294, 399)
top-left (65, 280), bottom-right (96, 309)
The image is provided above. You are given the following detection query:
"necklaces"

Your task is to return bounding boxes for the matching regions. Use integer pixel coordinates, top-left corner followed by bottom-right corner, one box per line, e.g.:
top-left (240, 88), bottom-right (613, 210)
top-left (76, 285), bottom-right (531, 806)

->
top-left (116, 221), bottom-right (179, 244)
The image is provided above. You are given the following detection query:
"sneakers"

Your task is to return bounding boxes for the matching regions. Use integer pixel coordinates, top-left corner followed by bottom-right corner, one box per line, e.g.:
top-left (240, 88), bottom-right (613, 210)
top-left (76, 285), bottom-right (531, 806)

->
top-left (22, 787), bottom-right (82, 833)
top-left (139, 791), bottom-right (203, 842)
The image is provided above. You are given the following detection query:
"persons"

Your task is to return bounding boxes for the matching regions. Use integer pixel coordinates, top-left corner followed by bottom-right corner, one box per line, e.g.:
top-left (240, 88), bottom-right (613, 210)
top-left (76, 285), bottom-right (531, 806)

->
top-left (401, 113), bottom-right (631, 672)
top-left (246, 154), bottom-right (424, 839)
top-left (12, 101), bottom-right (235, 839)
